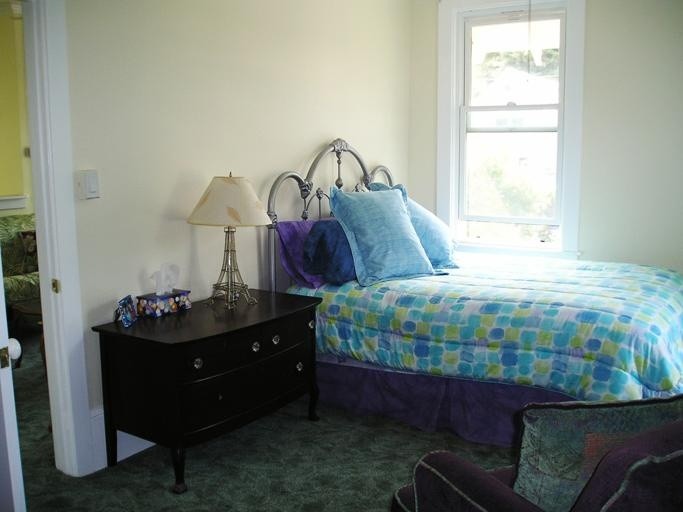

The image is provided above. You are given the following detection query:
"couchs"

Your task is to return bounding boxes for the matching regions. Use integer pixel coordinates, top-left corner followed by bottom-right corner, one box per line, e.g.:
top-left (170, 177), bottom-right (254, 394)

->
top-left (0, 212), bottom-right (42, 324)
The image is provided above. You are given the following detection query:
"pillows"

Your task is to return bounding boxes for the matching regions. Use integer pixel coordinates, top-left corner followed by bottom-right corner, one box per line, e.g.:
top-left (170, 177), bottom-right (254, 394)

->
top-left (328, 186), bottom-right (449, 287)
top-left (19, 230), bottom-right (38, 274)
top-left (369, 181), bottom-right (458, 269)
top-left (510, 393), bottom-right (683, 512)
top-left (304, 219), bottom-right (357, 286)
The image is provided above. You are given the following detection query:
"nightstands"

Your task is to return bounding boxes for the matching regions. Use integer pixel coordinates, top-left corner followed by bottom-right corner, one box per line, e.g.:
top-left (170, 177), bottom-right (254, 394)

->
top-left (91, 288), bottom-right (322, 493)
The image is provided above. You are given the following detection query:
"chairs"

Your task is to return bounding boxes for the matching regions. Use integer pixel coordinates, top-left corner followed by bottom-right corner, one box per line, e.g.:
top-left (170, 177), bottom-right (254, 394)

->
top-left (394, 420), bottom-right (683, 511)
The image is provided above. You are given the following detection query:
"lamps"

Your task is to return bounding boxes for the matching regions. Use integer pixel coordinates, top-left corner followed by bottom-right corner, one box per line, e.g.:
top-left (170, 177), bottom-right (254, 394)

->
top-left (187, 171), bottom-right (273, 311)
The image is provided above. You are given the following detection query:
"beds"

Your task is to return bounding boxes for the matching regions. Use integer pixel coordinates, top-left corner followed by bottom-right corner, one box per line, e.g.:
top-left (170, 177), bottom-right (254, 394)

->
top-left (266, 138), bottom-right (682, 449)
